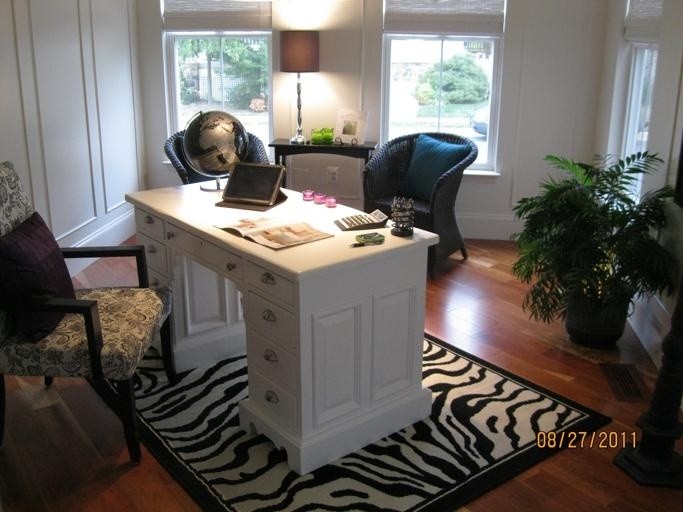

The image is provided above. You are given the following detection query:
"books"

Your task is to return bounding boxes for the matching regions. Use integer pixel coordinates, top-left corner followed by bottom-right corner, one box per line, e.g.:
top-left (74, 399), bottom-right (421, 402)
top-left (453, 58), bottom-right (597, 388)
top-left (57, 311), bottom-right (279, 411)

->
top-left (213, 215), bottom-right (334, 249)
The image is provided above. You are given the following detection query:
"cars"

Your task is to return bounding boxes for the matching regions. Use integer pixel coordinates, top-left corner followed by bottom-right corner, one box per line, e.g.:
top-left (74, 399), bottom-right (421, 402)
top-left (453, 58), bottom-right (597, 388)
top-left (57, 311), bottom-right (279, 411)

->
top-left (471, 105), bottom-right (489, 135)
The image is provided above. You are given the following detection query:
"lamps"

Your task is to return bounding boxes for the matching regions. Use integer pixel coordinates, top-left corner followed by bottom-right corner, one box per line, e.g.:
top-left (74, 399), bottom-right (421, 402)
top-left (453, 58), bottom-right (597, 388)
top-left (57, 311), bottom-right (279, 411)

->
top-left (280, 31), bottom-right (319, 142)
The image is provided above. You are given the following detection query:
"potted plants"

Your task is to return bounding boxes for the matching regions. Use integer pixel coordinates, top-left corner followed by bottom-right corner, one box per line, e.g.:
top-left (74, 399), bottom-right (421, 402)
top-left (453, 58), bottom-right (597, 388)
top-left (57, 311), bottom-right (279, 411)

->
top-left (509, 149), bottom-right (683, 348)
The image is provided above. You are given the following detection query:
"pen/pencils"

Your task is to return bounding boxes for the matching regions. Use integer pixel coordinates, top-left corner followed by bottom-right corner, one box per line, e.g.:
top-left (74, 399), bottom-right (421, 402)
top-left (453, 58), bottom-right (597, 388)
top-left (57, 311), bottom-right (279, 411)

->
top-left (351, 241), bottom-right (382, 247)
top-left (390, 196), bottom-right (413, 233)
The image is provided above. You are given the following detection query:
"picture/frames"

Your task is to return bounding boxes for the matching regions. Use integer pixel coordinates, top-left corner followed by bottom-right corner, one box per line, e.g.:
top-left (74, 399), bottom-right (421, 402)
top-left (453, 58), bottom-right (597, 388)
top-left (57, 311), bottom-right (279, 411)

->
top-left (333, 108), bottom-right (368, 144)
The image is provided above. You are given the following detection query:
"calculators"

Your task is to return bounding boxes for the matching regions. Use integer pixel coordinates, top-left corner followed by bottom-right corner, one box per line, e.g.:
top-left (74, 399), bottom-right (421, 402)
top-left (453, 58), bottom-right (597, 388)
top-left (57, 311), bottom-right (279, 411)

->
top-left (334, 209), bottom-right (388, 231)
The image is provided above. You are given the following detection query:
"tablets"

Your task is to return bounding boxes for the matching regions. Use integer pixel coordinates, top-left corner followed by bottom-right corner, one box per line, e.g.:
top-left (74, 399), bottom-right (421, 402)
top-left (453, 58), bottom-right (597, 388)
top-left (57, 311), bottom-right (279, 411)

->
top-left (223, 162), bottom-right (286, 205)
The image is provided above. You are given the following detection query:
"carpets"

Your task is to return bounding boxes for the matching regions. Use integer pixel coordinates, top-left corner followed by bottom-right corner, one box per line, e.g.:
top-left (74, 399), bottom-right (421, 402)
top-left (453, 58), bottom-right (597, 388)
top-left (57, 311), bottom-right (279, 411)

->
top-left (85, 331), bottom-right (614, 512)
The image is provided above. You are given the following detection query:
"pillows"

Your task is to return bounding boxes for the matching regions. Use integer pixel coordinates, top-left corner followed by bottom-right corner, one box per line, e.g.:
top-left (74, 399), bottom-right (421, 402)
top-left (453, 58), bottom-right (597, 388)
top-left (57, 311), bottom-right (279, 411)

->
top-left (0, 210), bottom-right (75, 342)
top-left (401, 133), bottom-right (471, 200)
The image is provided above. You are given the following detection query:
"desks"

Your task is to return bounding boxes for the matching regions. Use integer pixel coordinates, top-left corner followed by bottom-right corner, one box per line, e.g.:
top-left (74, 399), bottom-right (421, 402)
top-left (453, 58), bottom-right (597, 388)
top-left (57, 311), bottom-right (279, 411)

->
top-left (268, 138), bottom-right (378, 212)
top-left (125, 177), bottom-right (440, 476)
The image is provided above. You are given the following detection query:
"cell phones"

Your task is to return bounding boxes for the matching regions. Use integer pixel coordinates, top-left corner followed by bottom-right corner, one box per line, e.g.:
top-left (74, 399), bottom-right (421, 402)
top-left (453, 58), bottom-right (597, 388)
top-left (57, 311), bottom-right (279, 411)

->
top-left (356, 232), bottom-right (385, 243)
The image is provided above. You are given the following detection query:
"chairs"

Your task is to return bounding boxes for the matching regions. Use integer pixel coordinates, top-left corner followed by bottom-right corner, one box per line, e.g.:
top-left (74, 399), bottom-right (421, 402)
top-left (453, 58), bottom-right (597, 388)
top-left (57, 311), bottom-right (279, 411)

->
top-left (164, 130), bottom-right (270, 183)
top-left (0, 161), bottom-right (176, 463)
top-left (362, 132), bottom-right (478, 280)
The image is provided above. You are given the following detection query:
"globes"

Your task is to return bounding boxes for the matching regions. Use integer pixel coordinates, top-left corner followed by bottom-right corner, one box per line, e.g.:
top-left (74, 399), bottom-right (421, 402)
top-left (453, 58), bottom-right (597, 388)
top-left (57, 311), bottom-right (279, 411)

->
top-left (181, 111), bottom-right (248, 191)
